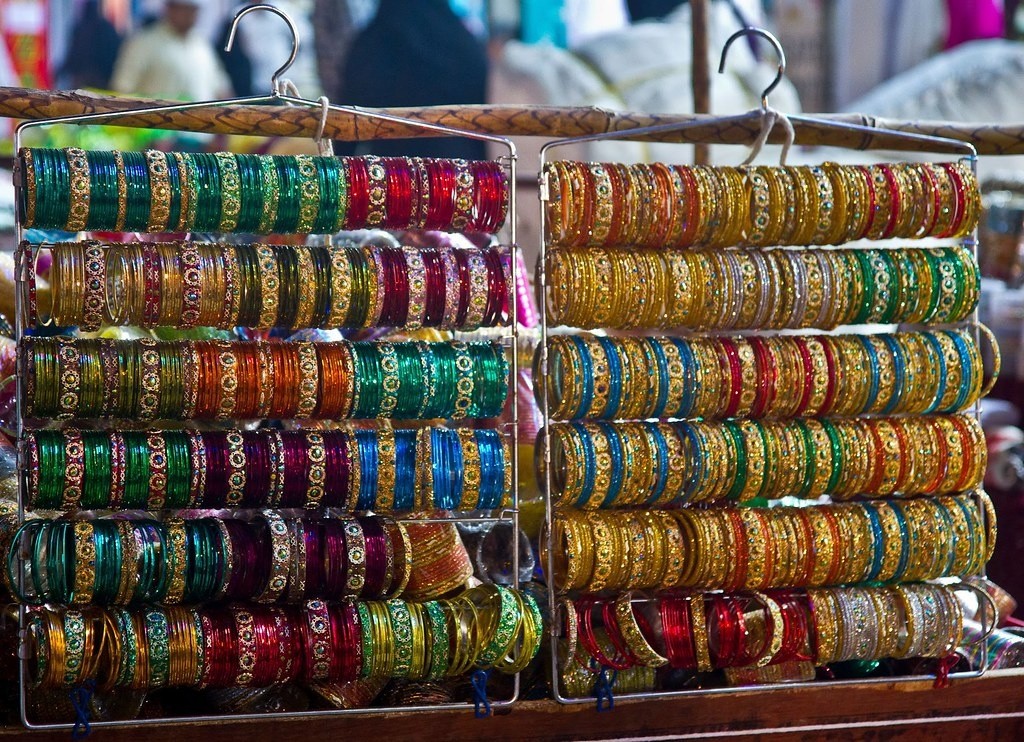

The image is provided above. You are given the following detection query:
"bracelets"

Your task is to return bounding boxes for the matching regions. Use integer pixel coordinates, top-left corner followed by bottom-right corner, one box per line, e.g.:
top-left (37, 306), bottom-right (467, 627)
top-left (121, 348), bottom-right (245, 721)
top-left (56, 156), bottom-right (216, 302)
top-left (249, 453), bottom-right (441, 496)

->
top-left (1, 112), bottom-right (543, 723)
top-left (532, 151), bottom-right (1023, 700)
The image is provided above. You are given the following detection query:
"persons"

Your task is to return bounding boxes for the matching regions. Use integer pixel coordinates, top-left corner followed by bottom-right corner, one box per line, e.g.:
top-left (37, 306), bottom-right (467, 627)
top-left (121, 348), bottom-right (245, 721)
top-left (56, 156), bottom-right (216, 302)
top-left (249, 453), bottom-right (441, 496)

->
top-left (50, 0), bottom-right (805, 149)
